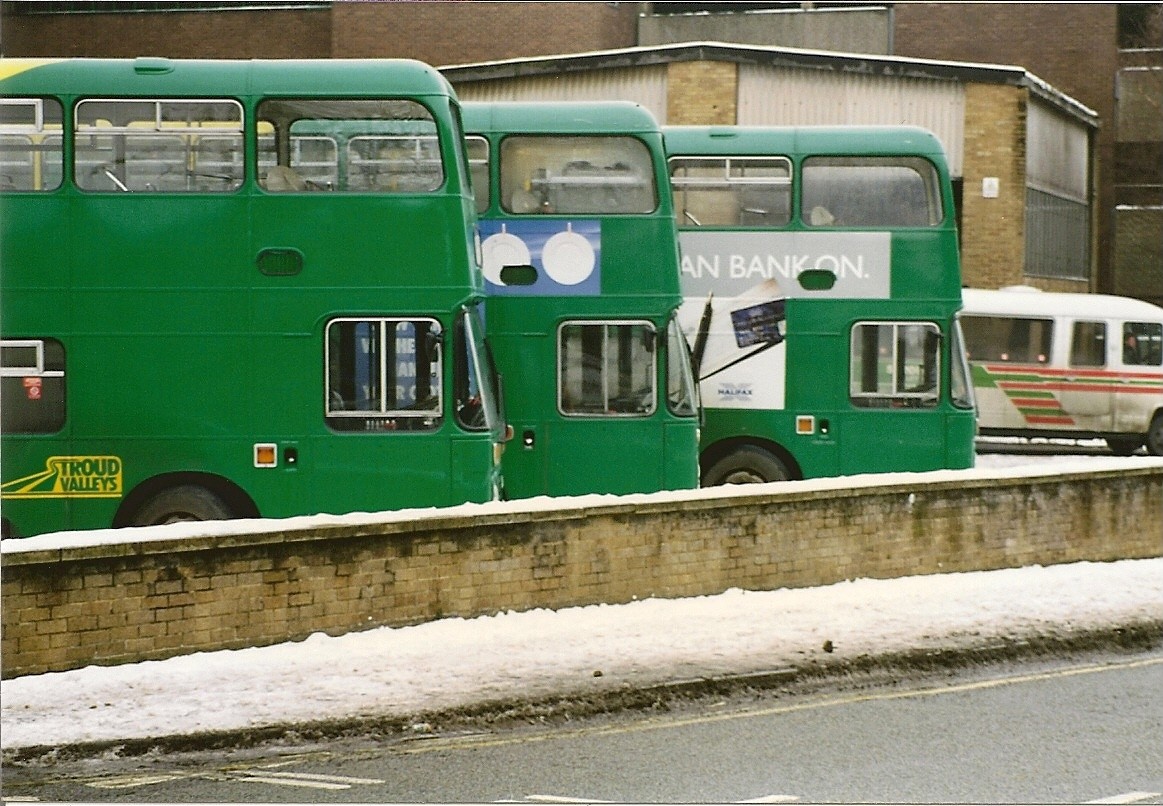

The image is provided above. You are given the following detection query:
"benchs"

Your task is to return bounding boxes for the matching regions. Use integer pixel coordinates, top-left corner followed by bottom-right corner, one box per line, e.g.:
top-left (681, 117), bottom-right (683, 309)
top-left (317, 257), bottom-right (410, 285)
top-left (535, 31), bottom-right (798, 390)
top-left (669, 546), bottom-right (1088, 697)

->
top-left (265, 165), bottom-right (340, 192)
top-left (95, 160), bottom-right (234, 191)
top-left (681, 202), bottom-right (812, 226)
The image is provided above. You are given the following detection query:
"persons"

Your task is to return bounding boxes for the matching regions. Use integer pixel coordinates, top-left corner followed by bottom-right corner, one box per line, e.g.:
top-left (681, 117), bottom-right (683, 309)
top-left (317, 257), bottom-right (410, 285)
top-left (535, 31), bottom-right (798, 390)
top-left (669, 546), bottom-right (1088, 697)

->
top-left (1123, 331), bottom-right (1149, 365)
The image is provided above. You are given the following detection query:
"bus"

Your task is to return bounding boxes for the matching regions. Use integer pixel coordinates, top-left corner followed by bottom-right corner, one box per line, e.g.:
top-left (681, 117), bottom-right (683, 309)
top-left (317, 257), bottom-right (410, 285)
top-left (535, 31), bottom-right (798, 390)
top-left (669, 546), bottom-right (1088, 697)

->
top-left (0, 58), bottom-right (979, 541)
top-left (851, 285), bottom-right (1163, 456)
